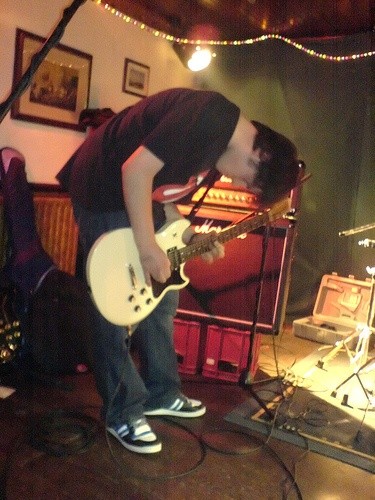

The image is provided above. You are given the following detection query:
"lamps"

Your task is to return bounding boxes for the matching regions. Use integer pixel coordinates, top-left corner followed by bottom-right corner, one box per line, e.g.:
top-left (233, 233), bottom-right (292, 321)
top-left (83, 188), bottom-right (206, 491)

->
top-left (170, 21), bottom-right (214, 73)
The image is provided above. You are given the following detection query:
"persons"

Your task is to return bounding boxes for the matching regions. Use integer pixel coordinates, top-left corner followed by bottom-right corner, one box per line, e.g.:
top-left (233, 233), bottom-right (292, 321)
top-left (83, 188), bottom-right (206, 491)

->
top-left (52, 87), bottom-right (299, 457)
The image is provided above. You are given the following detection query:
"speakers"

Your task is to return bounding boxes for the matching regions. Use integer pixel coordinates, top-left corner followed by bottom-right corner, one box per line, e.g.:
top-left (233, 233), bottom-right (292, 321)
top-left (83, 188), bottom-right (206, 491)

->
top-left (175, 204), bottom-right (297, 335)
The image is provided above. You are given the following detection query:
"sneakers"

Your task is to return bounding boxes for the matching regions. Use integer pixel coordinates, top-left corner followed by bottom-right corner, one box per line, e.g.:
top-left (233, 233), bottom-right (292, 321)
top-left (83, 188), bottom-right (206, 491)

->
top-left (143, 393), bottom-right (207, 417)
top-left (106, 416), bottom-right (162, 454)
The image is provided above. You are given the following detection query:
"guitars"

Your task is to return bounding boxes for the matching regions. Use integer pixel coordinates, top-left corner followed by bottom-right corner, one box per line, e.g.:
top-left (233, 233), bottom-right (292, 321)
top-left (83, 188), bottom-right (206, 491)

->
top-left (83, 195), bottom-right (293, 328)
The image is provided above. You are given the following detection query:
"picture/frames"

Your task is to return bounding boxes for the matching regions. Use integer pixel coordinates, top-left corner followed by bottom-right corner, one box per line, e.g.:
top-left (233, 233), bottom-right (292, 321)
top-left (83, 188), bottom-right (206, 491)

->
top-left (122, 57), bottom-right (151, 99)
top-left (10, 27), bottom-right (93, 133)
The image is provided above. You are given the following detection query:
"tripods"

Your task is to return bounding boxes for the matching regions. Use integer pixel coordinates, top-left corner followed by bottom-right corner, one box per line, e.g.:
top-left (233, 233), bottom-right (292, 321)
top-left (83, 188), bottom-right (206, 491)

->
top-left (181, 216), bottom-right (288, 429)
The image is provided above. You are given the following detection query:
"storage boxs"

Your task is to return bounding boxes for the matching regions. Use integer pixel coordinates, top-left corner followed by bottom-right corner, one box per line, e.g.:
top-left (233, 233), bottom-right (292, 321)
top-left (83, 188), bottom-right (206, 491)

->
top-left (200, 324), bottom-right (262, 384)
top-left (291, 271), bottom-right (375, 350)
top-left (171, 318), bottom-right (204, 378)
